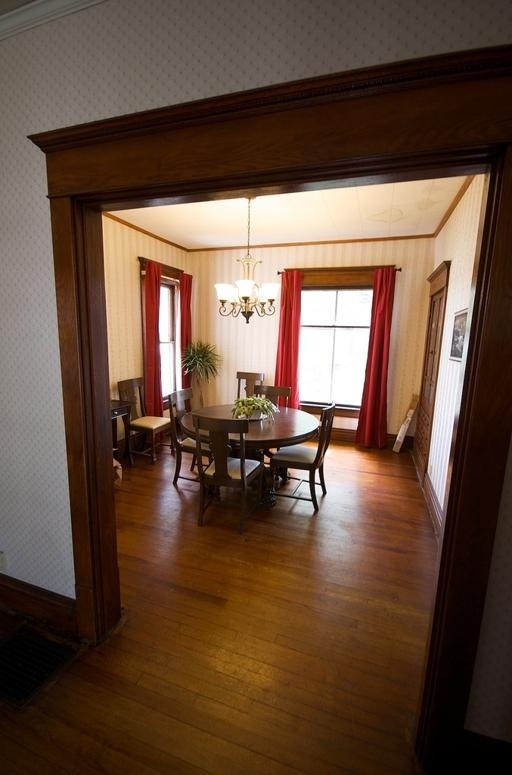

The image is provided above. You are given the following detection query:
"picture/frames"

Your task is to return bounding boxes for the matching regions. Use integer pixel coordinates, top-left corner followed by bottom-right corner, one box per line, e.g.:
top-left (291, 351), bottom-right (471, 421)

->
top-left (448, 308), bottom-right (469, 362)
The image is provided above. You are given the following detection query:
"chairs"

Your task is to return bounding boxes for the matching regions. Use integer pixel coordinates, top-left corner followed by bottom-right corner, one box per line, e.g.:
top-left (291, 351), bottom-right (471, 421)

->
top-left (117, 377), bottom-right (176, 465)
top-left (168, 372), bottom-right (335, 535)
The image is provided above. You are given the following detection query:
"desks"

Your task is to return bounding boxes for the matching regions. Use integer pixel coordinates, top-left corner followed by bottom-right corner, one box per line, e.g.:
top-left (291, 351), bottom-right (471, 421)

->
top-left (110, 400), bottom-right (135, 468)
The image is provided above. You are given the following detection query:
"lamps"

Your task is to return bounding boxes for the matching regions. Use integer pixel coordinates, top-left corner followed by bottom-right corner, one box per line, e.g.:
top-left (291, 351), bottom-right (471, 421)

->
top-left (214, 196), bottom-right (281, 323)
